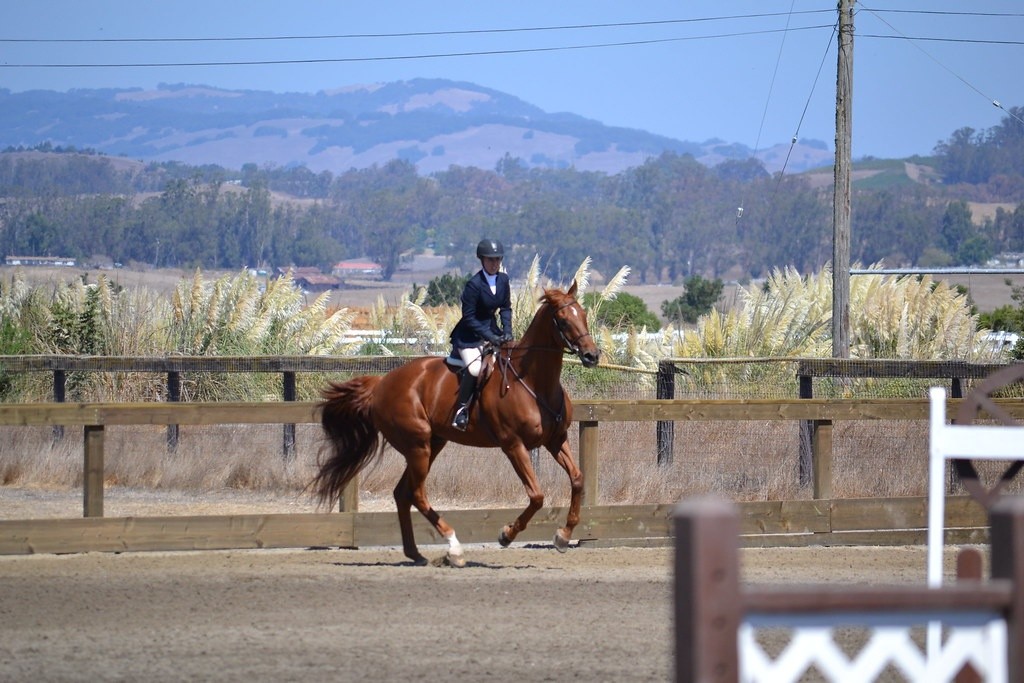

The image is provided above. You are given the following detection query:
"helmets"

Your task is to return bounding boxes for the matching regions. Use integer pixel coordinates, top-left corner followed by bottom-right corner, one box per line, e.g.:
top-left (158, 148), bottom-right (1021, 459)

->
top-left (476, 238), bottom-right (504, 258)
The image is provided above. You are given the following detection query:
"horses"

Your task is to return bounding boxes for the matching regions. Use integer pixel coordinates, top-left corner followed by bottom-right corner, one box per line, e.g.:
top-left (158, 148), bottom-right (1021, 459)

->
top-left (297, 278), bottom-right (600, 569)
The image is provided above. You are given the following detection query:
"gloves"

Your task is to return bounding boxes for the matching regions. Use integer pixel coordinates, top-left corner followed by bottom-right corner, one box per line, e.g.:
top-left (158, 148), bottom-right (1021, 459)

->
top-left (488, 335), bottom-right (504, 347)
top-left (501, 332), bottom-right (513, 342)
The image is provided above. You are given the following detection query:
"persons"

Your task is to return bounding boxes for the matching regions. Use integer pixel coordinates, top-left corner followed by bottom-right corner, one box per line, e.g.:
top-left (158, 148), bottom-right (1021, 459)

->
top-left (450, 238), bottom-right (514, 427)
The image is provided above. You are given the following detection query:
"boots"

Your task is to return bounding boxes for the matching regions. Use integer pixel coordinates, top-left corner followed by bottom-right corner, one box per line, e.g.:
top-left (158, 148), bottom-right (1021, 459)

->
top-left (456, 367), bottom-right (478, 427)
